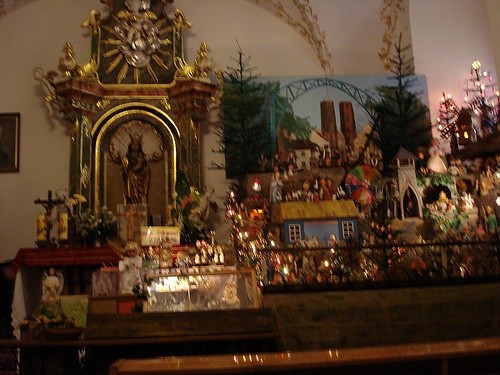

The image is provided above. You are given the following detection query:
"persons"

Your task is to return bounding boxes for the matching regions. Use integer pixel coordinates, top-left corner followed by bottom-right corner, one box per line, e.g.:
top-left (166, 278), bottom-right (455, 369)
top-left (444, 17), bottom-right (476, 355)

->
top-left (35, 81), bottom-right (498, 335)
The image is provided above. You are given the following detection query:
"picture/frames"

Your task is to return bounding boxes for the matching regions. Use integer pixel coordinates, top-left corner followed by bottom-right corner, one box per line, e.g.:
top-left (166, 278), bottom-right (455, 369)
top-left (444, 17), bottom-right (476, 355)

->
top-left (0, 112), bottom-right (21, 173)
